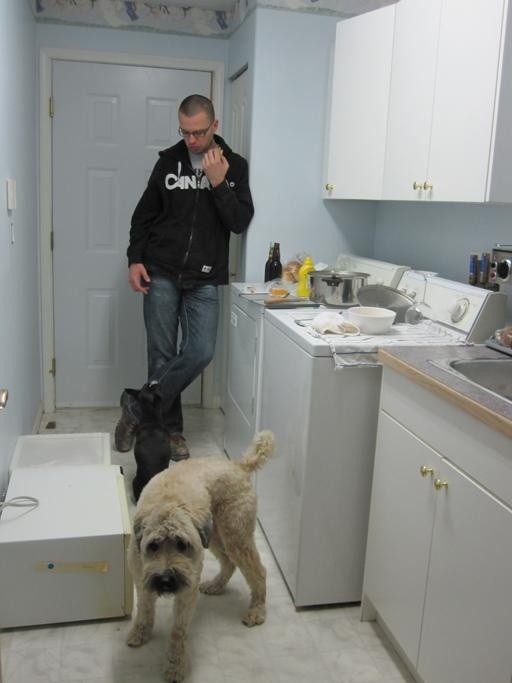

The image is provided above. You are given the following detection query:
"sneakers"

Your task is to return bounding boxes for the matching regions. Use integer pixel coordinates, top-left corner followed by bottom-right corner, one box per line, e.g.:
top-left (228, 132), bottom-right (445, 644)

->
top-left (168, 431), bottom-right (191, 460)
top-left (114, 391), bottom-right (139, 453)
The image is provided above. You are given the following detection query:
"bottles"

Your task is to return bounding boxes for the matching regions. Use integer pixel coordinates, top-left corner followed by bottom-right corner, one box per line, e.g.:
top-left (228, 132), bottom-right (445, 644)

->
top-left (298, 257), bottom-right (315, 297)
top-left (269, 242), bottom-right (282, 281)
top-left (265, 242), bottom-right (274, 283)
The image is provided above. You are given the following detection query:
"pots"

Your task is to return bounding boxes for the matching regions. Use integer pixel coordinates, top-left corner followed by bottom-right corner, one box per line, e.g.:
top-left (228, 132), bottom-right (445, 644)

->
top-left (307, 270), bottom-right (370, 306)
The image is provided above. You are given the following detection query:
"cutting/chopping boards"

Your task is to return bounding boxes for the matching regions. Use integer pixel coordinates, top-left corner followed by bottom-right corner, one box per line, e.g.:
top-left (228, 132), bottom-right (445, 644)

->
top-left (239, 292), bottom-right (321, 308)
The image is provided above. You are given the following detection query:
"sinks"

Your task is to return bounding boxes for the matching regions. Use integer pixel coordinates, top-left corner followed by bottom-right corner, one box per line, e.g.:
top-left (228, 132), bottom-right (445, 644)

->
top-left (424, 355), bottom-right (512, 405)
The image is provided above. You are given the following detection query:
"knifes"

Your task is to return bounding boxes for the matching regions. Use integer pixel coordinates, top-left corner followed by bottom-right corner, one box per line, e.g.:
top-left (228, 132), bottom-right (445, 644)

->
top-left (264, 297), bottom-right (307, 305)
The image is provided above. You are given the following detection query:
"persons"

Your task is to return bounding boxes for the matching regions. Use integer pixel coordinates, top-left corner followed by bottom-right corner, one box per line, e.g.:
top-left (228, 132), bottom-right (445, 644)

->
top-left (114, 94), bottom-right (254, 462)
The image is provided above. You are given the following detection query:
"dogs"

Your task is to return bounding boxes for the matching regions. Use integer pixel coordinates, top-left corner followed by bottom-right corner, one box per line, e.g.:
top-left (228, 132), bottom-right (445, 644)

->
top-left (127, 429), bottom-right (276, 682)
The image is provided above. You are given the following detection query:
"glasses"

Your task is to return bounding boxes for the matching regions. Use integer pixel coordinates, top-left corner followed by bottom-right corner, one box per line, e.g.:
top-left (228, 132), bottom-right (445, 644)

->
top-left (179, 125), bottom-right (213, 138)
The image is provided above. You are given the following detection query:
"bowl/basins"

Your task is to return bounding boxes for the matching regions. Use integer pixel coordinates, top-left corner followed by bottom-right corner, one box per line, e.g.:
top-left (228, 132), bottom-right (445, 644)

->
top-left (350, 306), bottom-right (397, 336)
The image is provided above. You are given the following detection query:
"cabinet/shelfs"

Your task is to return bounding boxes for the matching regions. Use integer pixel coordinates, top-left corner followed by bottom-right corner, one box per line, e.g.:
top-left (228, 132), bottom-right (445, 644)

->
top-left (321, 2), bottom-right (397, 203)
top-left (381, 0), bottom-right (512, 204)
top-left (360, 364), bottom-right (512, 682)
